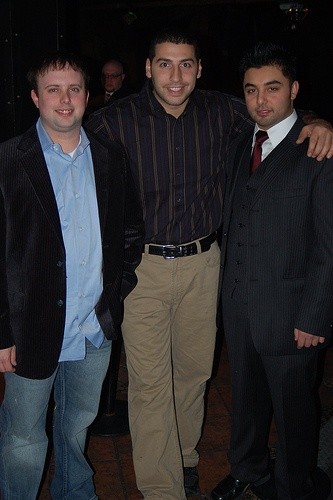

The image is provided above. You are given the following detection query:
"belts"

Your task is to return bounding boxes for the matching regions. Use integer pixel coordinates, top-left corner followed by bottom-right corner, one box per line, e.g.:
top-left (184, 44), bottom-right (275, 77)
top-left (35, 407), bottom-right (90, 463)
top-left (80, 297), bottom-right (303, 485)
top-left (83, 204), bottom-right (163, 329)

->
top-left (139, 233), bottom-right (218, 260)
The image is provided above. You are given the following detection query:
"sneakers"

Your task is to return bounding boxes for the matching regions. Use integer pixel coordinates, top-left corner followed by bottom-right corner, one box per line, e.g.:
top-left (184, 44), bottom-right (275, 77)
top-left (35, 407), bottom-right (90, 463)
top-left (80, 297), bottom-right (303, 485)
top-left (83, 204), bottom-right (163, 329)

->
top-left (183, 466), bottom-right (200, 497)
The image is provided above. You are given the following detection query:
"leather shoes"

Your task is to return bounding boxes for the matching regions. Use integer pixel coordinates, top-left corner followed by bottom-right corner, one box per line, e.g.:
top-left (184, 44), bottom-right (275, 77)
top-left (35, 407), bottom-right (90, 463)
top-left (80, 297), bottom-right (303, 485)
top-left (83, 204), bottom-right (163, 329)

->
top-left (209, 473), bottom-right (250, 500)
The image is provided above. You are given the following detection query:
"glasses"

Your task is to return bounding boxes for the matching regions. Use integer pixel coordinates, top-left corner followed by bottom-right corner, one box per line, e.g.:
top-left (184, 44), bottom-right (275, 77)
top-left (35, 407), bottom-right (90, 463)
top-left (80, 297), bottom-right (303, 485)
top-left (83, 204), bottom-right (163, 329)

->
top-left (103, 73), bottom-right (124, 79)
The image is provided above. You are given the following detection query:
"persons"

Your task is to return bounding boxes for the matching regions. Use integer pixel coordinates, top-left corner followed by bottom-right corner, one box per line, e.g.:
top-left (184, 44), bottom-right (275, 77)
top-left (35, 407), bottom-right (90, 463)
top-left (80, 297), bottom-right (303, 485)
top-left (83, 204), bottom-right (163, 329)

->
top-left (0, 48), bottom-right (145, 500)
top-left (78, 27), bottom-right (333, 499)
top-left (85, 60), bottom-right (132, 113)
top-left (208, 44), bottom-right (333, 500)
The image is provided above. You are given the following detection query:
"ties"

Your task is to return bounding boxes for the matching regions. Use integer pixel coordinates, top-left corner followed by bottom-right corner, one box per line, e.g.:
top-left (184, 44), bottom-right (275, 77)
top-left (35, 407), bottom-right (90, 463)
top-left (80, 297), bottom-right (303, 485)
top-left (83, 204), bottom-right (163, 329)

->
top-left (104, 94), bottom-right (111, 103)
top-left (250, 129), bottom-right (267, 174)
